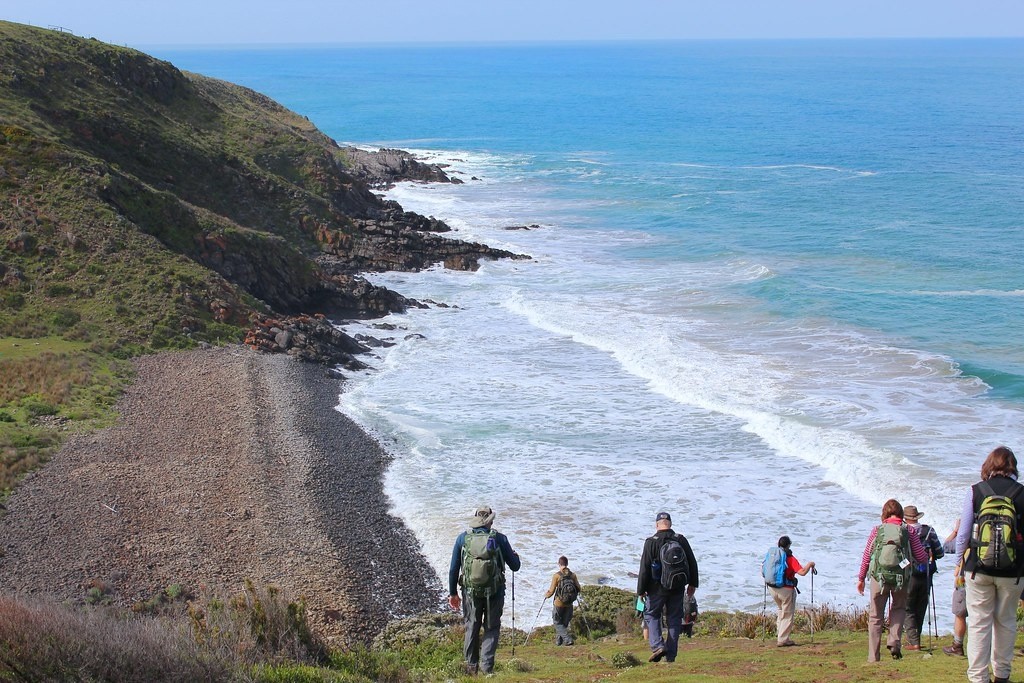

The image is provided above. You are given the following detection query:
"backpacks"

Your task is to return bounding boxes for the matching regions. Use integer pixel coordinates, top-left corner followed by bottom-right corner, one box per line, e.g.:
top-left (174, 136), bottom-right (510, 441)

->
top-left (866, 523), bottom-right (911, 593)
top-left (762, 545), bottom-right (795, 589)
top-left (685, 602), bottom-right (698, 624)
top-left (459, 528), bottom-right (505, 599)
top-left (969, 480), bottom-right (1024, 573)
top-left (646, 534), bottom-right (691, 590)
top-left (554, 571), bottom-right (577, 604)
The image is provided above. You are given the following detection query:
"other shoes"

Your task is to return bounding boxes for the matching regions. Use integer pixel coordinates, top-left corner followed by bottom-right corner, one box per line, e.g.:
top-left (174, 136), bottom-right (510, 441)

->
top-left (555, 642), bottom-right (562, 645)
top-left (778, 641), bottom-right (795, 647)
top-left (891, 648), bottom-right (904, 660)
top-left (483, 672), bottom-right (497, 677)
top-left (649, 648), bottom-right (667, 662)
top-left (943, 642), bottom-right (964, 657)
top-left (565, 642), bottom-right (574, 646)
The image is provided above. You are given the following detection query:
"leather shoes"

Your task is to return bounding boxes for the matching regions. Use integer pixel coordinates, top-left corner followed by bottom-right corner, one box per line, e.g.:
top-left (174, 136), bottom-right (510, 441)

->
top-left (902, 644), bottom-right (921, 651)
top-left (904, 636), bottom-right (912, 645)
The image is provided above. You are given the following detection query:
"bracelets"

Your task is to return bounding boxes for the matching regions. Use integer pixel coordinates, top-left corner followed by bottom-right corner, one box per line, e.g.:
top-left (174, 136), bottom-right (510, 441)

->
top-left (953, 530), bottom-right (957, 533)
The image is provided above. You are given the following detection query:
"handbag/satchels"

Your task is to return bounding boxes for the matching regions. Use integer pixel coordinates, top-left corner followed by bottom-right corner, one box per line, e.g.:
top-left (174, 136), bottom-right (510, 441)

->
top-left (910, 540), bottom-right (938, 575)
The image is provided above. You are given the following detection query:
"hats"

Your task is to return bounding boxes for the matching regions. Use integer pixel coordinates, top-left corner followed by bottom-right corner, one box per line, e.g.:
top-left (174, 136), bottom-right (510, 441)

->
top-left (902, 505), bottom-right (924, 520)
top-left (657, 512), bottom-right (670, 520)
top-left (468, 505), bottom-right (496, 528)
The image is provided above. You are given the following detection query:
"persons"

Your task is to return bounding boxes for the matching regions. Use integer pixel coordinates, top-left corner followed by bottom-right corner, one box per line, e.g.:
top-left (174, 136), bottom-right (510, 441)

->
top-left (767, 536), bottom-right (815, 646)
top-left (449, 505), bottom-right (520, 676)
top-left (902, 505), bottom-right (945, 651)
top-left (637, 512), bottom-right (699, 662)
top-left (681, 590), bottom-right (698, 638)
top-left (857, 499), bottom-right (929, 663)
top-left (545, 555), bottom-right (581, 646)
top-left (943, 519), bottom-right (968, 655)
top-left (641, 614), bottom-right (663, 640)
top-left (954, 445), bottom-right (1024, 683)
